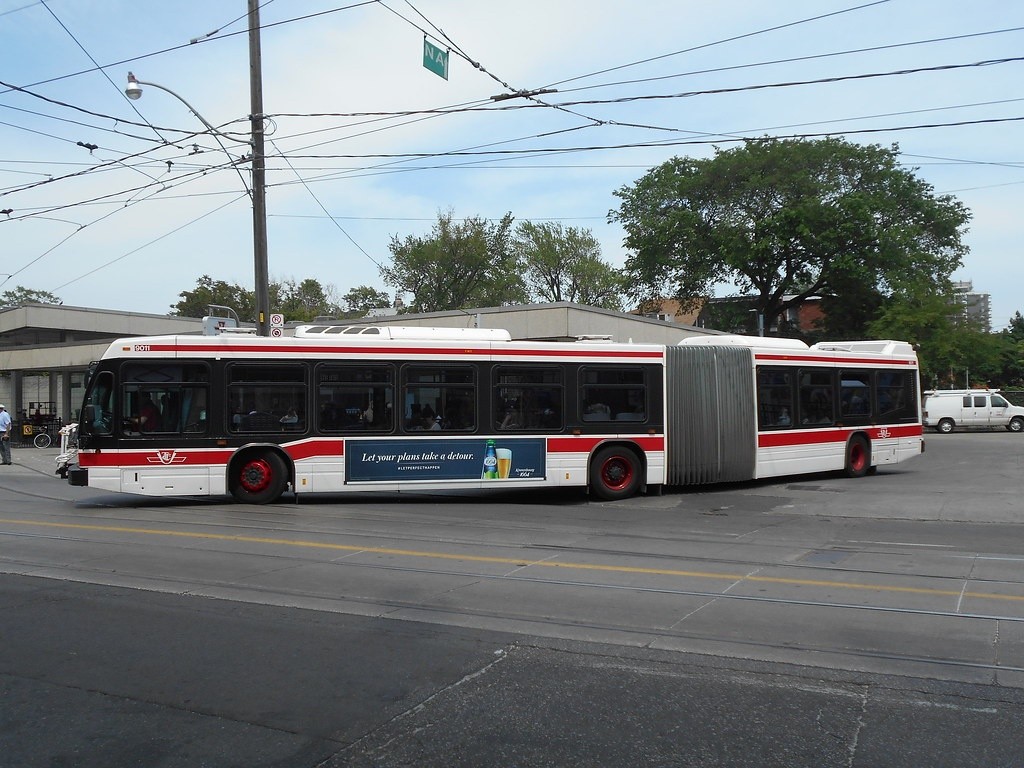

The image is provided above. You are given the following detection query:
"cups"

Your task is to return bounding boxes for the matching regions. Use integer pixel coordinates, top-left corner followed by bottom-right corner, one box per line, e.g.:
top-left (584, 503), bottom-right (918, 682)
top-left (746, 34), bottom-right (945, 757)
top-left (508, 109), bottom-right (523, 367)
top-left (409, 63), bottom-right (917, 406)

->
top-left (496, 449), bottom-right (511, 478)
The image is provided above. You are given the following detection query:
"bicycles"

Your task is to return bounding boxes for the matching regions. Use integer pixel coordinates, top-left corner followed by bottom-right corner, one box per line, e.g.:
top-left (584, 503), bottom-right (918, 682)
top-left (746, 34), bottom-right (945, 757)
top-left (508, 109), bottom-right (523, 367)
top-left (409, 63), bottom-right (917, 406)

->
top-left (33, 426), bottom-right (60, 449)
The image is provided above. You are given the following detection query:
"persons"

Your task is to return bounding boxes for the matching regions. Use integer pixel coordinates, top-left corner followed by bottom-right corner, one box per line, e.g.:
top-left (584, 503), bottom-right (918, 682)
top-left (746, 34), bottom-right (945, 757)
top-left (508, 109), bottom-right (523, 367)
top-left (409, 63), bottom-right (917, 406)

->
top-left (277, 404), bottom-right (831, 431)
top-left (124, 392), bottom-right (164, 432)
top-left (0, 404), bottom-right (13, 465)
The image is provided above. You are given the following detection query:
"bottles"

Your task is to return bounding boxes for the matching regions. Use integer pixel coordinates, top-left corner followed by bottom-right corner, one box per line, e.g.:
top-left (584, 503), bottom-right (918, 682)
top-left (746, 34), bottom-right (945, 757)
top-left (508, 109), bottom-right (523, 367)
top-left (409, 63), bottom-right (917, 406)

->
top-left (481, 440), bottom-right (499, 479)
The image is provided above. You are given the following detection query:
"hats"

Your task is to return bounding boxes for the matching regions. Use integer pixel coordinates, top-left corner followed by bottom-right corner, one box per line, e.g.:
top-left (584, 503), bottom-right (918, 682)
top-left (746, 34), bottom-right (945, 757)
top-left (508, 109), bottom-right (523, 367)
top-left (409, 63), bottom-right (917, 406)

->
top-left (0, 403), bottom-right (5, 407)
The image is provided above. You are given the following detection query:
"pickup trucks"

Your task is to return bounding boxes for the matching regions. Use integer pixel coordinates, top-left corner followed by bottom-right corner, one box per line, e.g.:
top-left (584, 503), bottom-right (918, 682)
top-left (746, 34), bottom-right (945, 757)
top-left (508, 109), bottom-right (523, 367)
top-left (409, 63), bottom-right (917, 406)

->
top-left (923, 389), bottom-right (1024, 433)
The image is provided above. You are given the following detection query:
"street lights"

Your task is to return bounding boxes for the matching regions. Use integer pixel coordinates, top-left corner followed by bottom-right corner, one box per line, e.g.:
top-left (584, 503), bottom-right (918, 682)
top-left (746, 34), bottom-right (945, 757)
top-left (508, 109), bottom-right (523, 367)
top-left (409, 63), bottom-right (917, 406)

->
top-left (124, 69), bottom-right (271, 333)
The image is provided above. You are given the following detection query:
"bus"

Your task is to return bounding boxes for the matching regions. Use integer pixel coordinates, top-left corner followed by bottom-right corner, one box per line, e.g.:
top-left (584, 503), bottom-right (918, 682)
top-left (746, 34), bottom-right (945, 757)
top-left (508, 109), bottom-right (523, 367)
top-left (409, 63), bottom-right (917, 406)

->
top-left (55, 319), bottom-right (929, 504)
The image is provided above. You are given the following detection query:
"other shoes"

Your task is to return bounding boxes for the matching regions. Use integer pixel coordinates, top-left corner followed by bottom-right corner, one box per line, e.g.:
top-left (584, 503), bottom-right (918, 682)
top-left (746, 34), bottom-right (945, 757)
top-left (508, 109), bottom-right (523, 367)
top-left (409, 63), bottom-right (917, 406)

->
top-left (0, 462), bottom-right (7, 465)
top-left (7, 462), bottom-right (12, 465)
